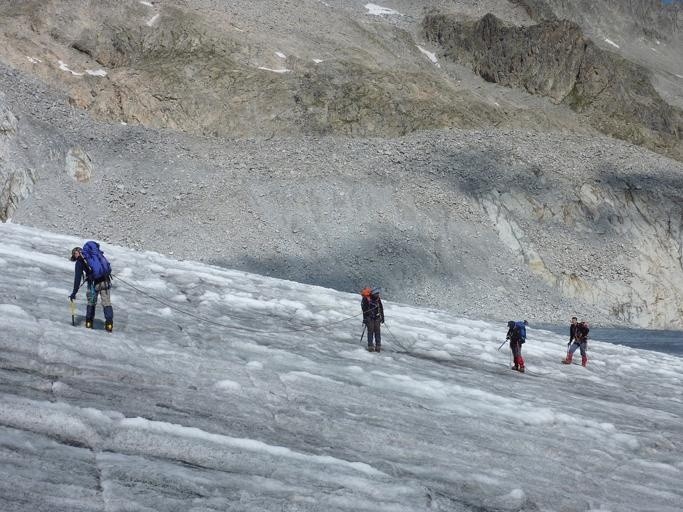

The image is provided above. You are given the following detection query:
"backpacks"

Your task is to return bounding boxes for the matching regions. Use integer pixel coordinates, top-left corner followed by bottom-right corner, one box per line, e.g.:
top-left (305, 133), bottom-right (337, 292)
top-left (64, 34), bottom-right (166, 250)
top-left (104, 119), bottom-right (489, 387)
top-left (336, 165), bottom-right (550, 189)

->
top-left (82, 241), bottom-right (112, 280)
top-left (515, 321), bottom-right (525, 344)
top-left (582, 321), bottom-right (590, 340)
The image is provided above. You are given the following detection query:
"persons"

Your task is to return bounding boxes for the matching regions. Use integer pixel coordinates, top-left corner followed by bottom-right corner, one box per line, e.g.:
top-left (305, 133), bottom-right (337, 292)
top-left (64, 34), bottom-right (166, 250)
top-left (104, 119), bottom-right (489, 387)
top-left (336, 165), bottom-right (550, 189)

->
top-left (561, 316), bottom-right (589, 367)
top-left (506, 321), bottom-right (525, 372)
top-left (69, 247), bottom-right (114, 332)
top-left (360, 288), bottom-right (385, 353)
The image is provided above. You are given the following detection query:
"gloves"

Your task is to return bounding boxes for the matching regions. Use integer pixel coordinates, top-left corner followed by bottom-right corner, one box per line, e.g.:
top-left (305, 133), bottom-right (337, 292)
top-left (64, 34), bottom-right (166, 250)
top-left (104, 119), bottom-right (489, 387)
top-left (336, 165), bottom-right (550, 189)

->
top-left (68, 293), bottom-right (76, 303)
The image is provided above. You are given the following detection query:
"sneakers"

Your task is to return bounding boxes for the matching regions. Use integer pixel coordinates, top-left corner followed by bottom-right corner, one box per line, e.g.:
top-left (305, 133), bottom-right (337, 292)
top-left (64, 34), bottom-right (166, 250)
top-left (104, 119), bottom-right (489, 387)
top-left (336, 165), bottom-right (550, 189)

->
top-left (512, 365), bottom-right (519, 370)
top-left (520, 368), bottom-right (524, 372)
top-left (368, 347), bottom-right (373, 351)
top-left (561, 360), bottom-right (569, 364)
top-left (106, 324), bottom-right (113, 331)
top-left (375, 347), bottom-right (381, 352)
top-left (86, 320), bottom-right (94, 328)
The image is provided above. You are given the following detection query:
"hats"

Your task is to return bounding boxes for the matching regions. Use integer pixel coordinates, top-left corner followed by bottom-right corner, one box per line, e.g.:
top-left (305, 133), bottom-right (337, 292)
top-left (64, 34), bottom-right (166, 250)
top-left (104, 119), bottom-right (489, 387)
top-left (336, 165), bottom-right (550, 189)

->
top-left (362, 287), bottom-right (371, 296)
top-left (70, 247), bottom-right (80, 260)
top-left (507, 321), bottom-right (513, 327)
